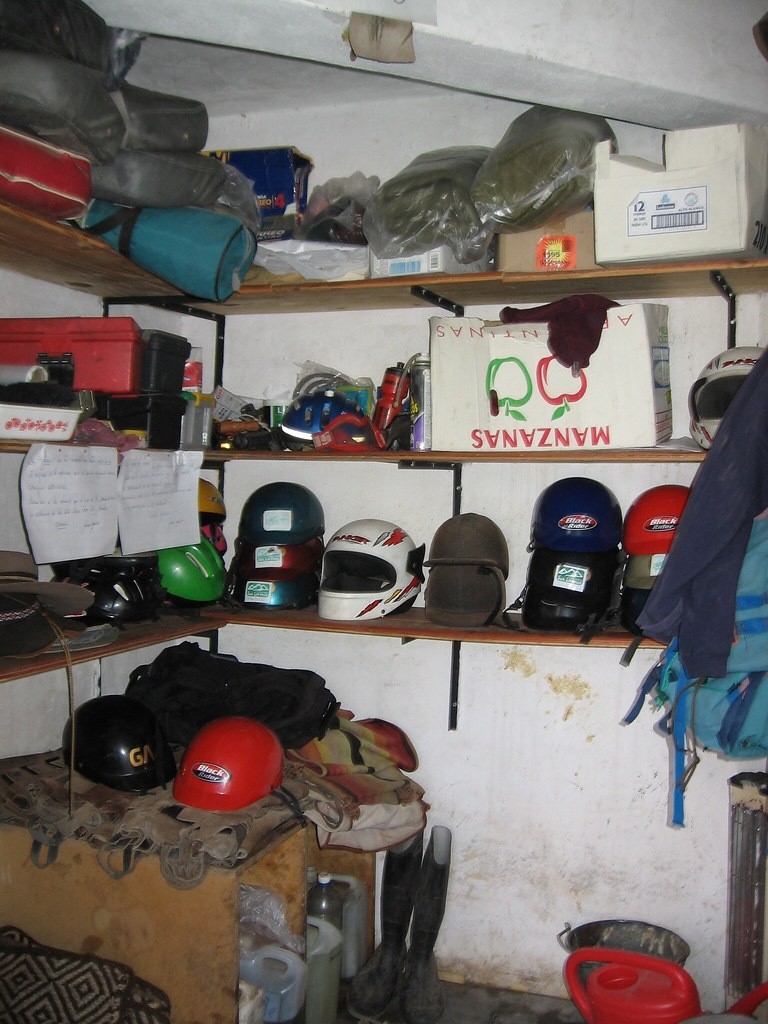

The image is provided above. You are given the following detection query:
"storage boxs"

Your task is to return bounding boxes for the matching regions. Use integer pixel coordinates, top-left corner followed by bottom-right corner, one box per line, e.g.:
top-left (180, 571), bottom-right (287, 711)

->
top-left (200, 144), bottom-right (315, 244)
top-left (182, 347), bottom-right (203, 393)
top-left (180, 393), bottom-right (216, 450)
top-left (254, 240), bottom-right (372, 284)
top-left (370, 239), bottom-right (485, 280)
top-left (499, 211), bottom-right (605, 271)
top-left (431, 303), bottom-right (673, 453)
top-left (140, 329), bottom-right (192, 397)
top-left (1, 317), bottom-right (143, 398)
top-left (594, 123), bottom-right (768, 265)
top-left (94, 395), bottom-right (186, 452)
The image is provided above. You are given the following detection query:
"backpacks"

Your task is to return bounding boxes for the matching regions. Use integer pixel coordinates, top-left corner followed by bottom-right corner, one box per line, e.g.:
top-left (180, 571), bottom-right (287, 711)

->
top-left (121, 641), bottom-right (341, 746)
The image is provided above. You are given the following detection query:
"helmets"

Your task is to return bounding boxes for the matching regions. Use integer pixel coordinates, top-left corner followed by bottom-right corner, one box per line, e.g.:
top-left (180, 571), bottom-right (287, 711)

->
top-left (172, 715), bottom-right (284, 812)
top-left (690, 346), bottom-right (766, 449)
top-left (64, 695), bottom-right (175, 792)
top-left (78, 475), bottom-right (696, 639)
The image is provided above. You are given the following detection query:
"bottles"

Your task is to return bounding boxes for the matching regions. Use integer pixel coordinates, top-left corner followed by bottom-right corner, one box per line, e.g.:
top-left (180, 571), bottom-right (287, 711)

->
top-left (307, 866), bottom-right (368, 979)
top-left (410, 352), bottom-right (433, 453)
top-left (306, 916), bottom-right (343, 1023)
top-left (308, 869), bottom-right (342, 935)
top-left (371, 363), bottom-right (409, 431)
top-left (239, 948), bottom-right (307, 1024)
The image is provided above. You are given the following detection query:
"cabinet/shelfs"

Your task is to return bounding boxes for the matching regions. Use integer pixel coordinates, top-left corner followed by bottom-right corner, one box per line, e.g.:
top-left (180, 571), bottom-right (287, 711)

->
top-left (0, 809), bottom-right (377, 1024)
top-left (0, 198), bottom-right (767, 683)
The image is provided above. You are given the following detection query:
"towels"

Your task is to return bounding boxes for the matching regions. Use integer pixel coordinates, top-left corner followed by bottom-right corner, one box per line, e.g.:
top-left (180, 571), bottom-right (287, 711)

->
top-left (498, 293), bottom-right (625, 377)
